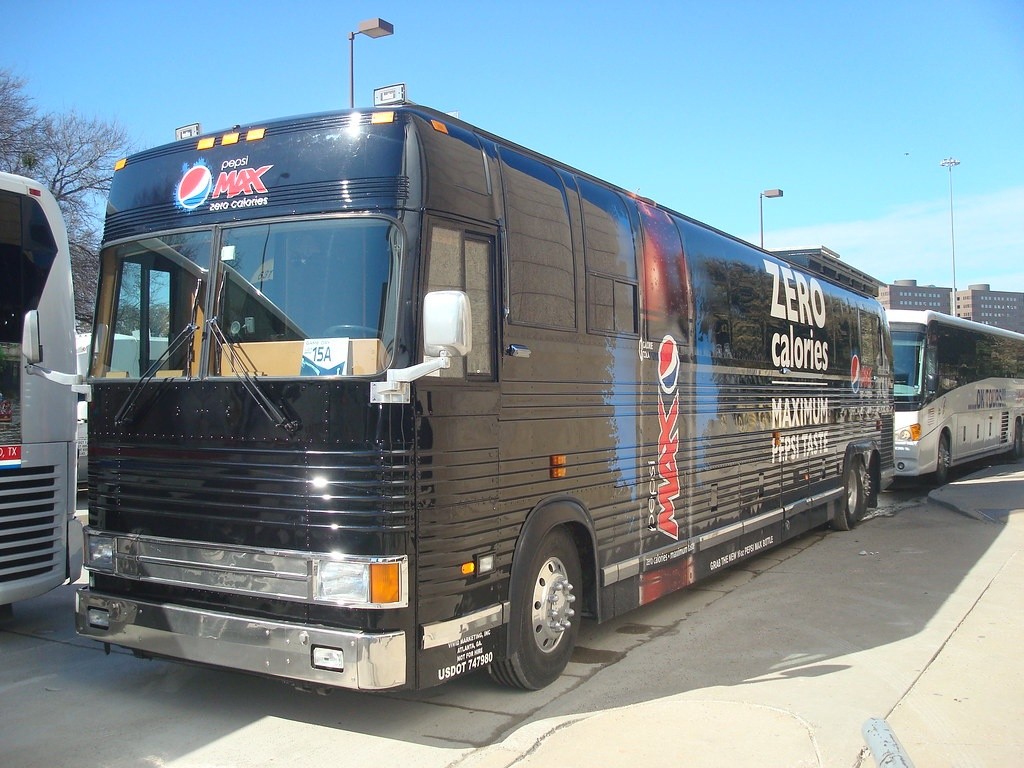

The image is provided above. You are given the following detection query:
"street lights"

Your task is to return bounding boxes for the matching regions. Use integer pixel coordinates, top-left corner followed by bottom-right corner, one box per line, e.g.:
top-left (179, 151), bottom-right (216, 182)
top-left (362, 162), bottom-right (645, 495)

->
top-left (349, 17), bottom-right (393, 107)
top-left (760, 187), bottom-right (785, 249)
top-left (941, 156), bottom-right (961, 317)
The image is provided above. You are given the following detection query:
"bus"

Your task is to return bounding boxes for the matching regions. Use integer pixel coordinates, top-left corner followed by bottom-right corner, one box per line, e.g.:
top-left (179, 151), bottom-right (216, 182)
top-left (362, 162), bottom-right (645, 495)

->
top-left (0, 169), bottom-right (82, 619)
top-left (69, 78), bottom-right (899, 700)
top-left (883, 308), bottom-right (1024, 485)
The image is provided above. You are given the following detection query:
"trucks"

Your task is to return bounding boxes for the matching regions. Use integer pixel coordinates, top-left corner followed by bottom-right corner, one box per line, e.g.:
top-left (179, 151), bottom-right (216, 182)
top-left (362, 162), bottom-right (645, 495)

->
top-left (77, 331), bottom-right (169, 483)
